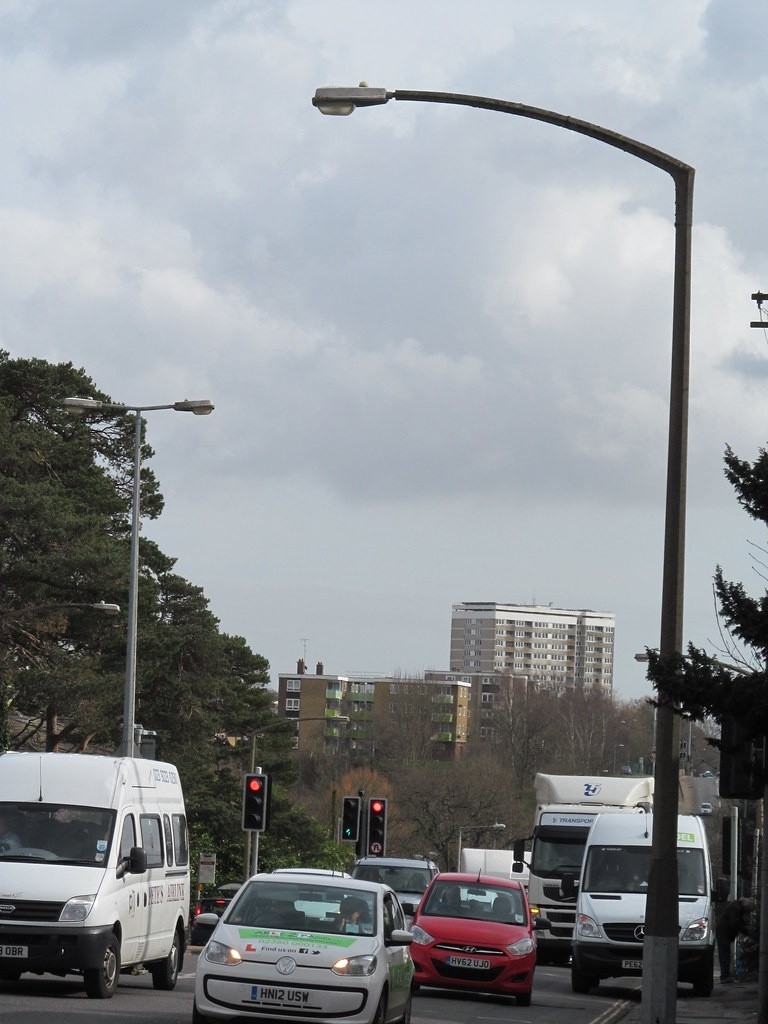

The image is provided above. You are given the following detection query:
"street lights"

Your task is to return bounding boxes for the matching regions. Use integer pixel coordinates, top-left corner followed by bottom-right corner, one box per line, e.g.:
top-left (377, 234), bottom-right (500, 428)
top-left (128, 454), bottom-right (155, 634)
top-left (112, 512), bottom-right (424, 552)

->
top-left (312, 80), bottom-right (695, 1024)
top-left (457, 824), bottom-right (506, 873)
top-left (633, 652), bottom-right (764, 842)
top-left (63, 396), bottom-right (215, 758)
top-left (243, 716), bottom-right (351, 885)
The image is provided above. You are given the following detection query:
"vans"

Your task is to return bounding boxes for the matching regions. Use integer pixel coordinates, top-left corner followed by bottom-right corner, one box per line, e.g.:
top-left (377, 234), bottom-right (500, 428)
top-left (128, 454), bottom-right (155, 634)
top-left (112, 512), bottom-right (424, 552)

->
top-left (351, 857), bottom-right (440, 929)
top-left (560, 811), bottom-right (730, 999)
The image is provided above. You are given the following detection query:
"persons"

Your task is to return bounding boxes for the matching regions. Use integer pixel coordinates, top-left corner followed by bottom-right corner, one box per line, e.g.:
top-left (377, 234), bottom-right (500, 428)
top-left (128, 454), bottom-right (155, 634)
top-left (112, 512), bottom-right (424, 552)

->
top-left (362, 868), bottom-right (382, 883)
top-left (430, 886), bottom-right (465, 914)
top-left (270, 901), bottom-right (303, 930)
top-left (328, 896), bottom-right (365, 933)
top-left (0, 814), bottom-right (24, 853)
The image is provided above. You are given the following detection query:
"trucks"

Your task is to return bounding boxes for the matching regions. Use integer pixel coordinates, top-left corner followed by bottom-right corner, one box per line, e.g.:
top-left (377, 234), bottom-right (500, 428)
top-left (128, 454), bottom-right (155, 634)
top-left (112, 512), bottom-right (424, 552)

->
top-left (461, 848), bottom-right (533, 894)
top-left (506, 773), bottom-right (656, 965)
top-left (0, 751), bottom-right (191, 999)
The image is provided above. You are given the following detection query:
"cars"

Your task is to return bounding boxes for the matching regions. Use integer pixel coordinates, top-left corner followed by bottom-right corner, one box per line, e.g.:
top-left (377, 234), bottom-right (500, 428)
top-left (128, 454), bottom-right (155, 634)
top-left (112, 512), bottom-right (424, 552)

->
top-left (401, 871), bottom-right (552, 1007)
top-left (698, 803), bottom-right (714, 815)
top-left (191, 867), bottom-right (416, 1024)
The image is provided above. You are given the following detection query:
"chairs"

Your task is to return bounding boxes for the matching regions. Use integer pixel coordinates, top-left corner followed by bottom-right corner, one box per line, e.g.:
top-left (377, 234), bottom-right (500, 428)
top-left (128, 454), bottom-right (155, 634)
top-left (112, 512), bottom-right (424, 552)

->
top-left (274, 900), bottom-right (306, 921)
top-left (441, 888), bottom-right (470, 915)
top-left (480, 896), bottom-right (516, 920)
top-left (677, 862), bottom-right (698, 894)
top-left (253, 912), bottom-right (285, 929)
top-left (53, 820), bottom-right (106, 863)
top-left (407, 872), bottom-right (427, 891)
top-left (339, 897), bottom-right (371, 923)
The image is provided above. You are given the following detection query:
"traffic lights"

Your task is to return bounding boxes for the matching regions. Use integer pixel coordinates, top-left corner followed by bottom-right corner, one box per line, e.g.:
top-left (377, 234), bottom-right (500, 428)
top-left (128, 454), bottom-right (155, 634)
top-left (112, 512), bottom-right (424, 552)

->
top-left (241, 774), bottom-right (268, 833)
top-left (339, 796), bottom-right (361, 843)
top-left (366, 797), bottom-right (387, 857)
